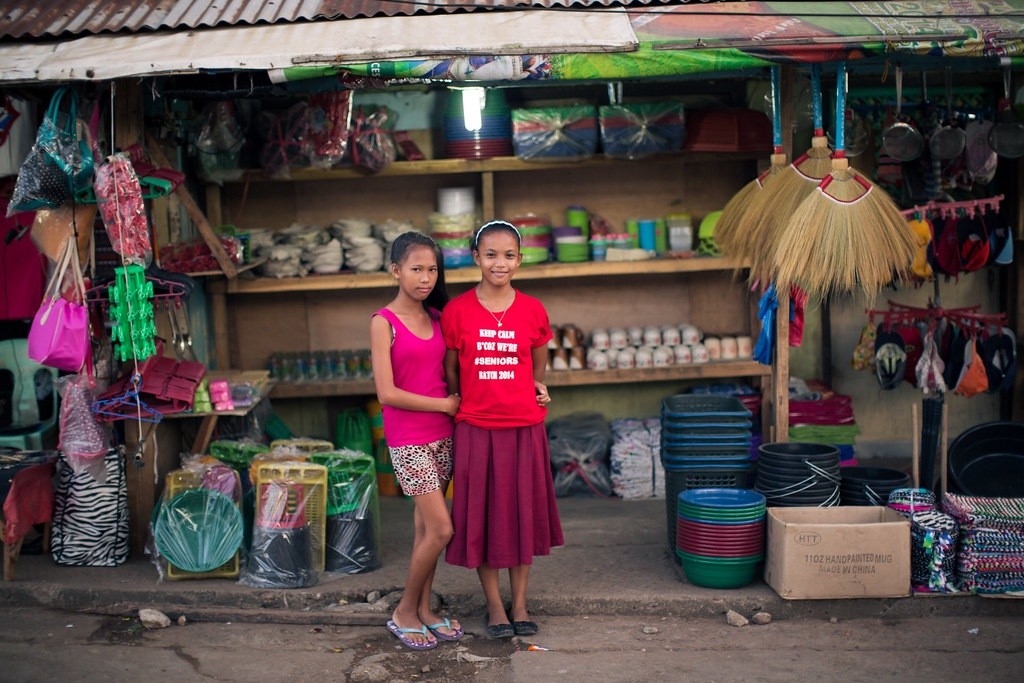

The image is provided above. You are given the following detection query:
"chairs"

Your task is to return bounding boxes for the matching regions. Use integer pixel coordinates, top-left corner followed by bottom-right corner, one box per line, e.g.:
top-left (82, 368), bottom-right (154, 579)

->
top-left (0, 338), bottom-right (58, 452)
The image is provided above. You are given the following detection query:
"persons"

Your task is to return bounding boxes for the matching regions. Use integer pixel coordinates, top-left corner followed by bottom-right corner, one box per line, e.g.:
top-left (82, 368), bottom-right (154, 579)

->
top-left (369, 230), bottom-right (552, 651)
top-left (440, 219), bottom-right (566, 639)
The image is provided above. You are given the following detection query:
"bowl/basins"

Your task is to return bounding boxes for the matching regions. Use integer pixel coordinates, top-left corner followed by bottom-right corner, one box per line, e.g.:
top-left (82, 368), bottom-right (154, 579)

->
top-left (513, 219), bottom-right (588, 263)
top-left (933, 477), bottom-right (964, 512)
top-left (949, 420), bottom-right (1024, 501)
top-left (235, 219), bottom-right (424, 276)
top-left (155, 489), bottom-right (243, 572)
top-left (430, 214), bottom-right (476, 268)
top-left (444, 87), bottom-right (512, 157)
top-left (677, 489), bottom-right (765, 588)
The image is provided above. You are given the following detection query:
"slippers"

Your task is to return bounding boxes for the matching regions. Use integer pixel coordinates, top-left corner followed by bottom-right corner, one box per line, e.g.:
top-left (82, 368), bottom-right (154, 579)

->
top-left (427, 617), bottom-right (464, 641)
top-left (387, 620), bottom-right (437, 650)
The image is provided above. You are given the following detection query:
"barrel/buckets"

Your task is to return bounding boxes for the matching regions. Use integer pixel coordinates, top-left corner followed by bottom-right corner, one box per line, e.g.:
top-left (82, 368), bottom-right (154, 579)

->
top-left (756, 443), bottom-right (908, 505)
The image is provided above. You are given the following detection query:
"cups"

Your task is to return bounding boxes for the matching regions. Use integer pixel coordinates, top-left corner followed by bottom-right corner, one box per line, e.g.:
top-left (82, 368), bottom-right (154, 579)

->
top-left (545, 324), bottom-right (752, 370)
top-left (637, 221), bottom-right (655, 250)
top-left (626, 220), bottom-right (640, 249)
top-left (654, 220), bottom-right (667, 251)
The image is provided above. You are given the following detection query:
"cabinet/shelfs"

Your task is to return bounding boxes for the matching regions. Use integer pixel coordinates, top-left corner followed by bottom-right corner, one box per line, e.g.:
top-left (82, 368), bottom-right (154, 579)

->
top-left (200, 72), bottom-right (789, 443)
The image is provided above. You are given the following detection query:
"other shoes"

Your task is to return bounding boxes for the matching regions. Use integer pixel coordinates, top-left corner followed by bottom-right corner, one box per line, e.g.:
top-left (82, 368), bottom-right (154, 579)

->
top-left (486, 612), bottom-right (515, 638)
top-left (508, 608), bottom-right (538, 635)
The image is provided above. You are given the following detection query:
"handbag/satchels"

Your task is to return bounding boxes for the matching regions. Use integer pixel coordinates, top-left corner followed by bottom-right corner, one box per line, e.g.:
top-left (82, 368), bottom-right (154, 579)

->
top-left (9, 88), bottom-right (94, 211)
top-left (57, 374), bottom-right (108, 458)
top-left (28, 236), bottom-right (89, 372)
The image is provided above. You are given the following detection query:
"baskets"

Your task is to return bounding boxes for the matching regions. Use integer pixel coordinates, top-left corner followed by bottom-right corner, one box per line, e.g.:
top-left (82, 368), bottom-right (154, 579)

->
top-left (660, 393), bottom-right (753, 568)
top-left (310, 453), bottom-right (380, 556)
top-left (256, 464), bottom-right (328, 573)
top-left (166, 469), bottom-right (242, 580)
top-left (271, 439), bottom-right (333, 459)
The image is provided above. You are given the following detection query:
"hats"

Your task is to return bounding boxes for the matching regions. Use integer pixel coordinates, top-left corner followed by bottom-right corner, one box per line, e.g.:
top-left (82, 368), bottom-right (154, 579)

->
top-left (915, 320), bottom-right (1017, 398)
top-left (984, 209), bottom-right (1013, 268)
top-left (932, 214), bottom-right (961, 285)
top-left (850, 322), bottom-right (876, 370)
top-left (877, 323), bottom-right (898, 331)
top-left (956, 215), bottom-right (990, 272)
top-left (874, 331), bottom-right (907, 390)
top-left (909, 211), bottom-right (933, 277)
top-left (896, 325), bottom-right (922, 385)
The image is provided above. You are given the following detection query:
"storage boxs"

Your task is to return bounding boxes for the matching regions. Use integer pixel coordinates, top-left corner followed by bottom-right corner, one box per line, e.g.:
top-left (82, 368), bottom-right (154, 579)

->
top-left (764, 506), bottom-right (910, 600)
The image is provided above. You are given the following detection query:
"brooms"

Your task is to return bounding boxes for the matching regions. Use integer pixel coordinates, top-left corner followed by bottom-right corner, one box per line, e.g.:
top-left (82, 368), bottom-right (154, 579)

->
top-left (711, 62), bottom-right (921, 318)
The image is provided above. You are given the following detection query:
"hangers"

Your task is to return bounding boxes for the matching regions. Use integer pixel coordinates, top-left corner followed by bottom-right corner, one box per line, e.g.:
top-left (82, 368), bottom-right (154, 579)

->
top-left (74, 143), bottom-right (186, 203)
top-left (91, 338), bottom-right (207, 424)
top-left (83, 253), bottom-right (196, 313)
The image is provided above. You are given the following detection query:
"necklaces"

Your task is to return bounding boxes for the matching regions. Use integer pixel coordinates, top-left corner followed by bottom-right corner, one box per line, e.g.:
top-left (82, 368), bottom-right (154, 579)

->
top-left (478, 283), bottom-right (514, 327)
